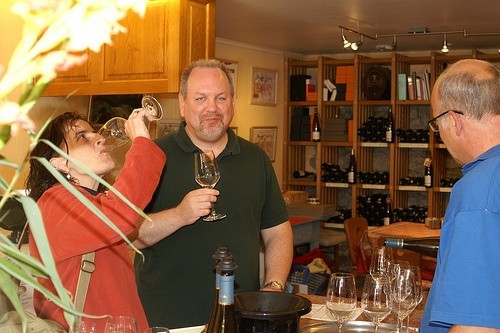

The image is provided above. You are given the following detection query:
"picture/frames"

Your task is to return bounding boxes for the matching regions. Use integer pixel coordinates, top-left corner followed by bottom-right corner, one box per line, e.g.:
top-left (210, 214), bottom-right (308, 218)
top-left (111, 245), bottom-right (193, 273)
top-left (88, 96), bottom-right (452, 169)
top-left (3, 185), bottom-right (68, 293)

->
top-left (215, 57), bottom-right (240, 102)
top-left (250, 67), bottom-right (278, 107)
top-left (156, 118), bottom-right (182, 140)
top-left (250, 125), bottom-right (277, 164)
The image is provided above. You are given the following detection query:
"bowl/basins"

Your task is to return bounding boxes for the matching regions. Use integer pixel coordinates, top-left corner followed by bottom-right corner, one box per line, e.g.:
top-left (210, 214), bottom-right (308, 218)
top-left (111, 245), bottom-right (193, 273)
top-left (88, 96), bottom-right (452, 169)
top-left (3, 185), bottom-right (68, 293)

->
top-left (230, 291), bottom-right (312, 333)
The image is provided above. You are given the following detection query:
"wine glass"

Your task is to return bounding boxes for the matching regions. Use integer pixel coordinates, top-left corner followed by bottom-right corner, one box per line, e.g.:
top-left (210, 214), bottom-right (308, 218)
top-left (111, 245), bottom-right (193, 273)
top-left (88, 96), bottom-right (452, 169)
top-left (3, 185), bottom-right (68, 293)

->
top-left (94, 95), bottom-right (163, 152)
top-left (360, 247), bottom-right (424, 333)
top-left (194, 150), bottom-right (226, 221)
top-left (325, 273), bottom-right (357, 333)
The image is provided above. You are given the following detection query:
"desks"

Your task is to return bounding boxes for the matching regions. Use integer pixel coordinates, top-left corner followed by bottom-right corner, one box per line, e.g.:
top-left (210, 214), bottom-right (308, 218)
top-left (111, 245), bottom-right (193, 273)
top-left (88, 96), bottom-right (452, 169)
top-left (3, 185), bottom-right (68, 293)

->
top-left (366, 221), bottom-right (441, 271)
top-left (287, 210), bottom-right (341, 254)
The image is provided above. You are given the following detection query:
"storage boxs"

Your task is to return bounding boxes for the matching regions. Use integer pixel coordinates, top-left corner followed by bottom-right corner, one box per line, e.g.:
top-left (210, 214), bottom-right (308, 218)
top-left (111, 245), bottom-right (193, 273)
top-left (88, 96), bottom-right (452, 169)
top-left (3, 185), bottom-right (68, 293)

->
top-left (281, 190), bottom-right (309, 207)
top-left (290, 202), bottom-right (336, 217)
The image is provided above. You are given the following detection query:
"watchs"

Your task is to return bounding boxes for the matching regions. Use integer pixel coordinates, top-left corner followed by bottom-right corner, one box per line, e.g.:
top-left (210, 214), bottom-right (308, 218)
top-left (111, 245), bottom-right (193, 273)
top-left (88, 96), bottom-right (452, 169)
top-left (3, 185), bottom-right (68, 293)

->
top-left (263, 281), bottom-right (282, 290)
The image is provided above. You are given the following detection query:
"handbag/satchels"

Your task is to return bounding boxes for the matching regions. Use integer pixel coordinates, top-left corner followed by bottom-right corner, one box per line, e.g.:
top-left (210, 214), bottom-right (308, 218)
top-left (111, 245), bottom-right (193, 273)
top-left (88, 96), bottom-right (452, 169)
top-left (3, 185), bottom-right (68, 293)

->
top-left (0, 307), bottom-right (76, 333)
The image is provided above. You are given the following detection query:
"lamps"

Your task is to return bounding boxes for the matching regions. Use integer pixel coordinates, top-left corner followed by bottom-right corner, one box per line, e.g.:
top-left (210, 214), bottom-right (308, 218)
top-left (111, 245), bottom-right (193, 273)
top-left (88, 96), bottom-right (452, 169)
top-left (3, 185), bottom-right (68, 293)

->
top-left (350, 34), bottom-right (364, 50)
top-left (442, 33), bottom-right (450, 53)
top-left (341, 28), bottom-right (351, 48)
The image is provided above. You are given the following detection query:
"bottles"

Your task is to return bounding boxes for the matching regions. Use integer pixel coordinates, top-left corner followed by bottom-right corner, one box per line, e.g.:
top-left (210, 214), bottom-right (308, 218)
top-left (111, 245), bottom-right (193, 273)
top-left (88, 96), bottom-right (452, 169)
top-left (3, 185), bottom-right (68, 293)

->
top-left (360, 195), bottom-right (393, 226)
top-left (395, 205), bottom-right (428, 224)
top-left (386, 106), bottom-right (394, 143)
top-left (440, 178), bottom-right (460, 187)
top-left (400, 176), bottom-right (424, 186)
top-left (335, 209), bottom-right (351, 220)
top-left (321, 163), bottom-right (349, 183)
top-left (348, 149), bottom-right (356, 184)
top-left (424, 151), bottom-right (432, 188)
top-left (293, 171), bottom-right (314, 178)
top-left (384, 237), bottom-right (439, 258)
top-left (201, 246), bottom-right (238, 333)
top-left (312, 108), bottom-right (321, 142)
top-left (397, 128), bottom-right (430, 144)
top-left (358, 116), bottom-right (387, 142)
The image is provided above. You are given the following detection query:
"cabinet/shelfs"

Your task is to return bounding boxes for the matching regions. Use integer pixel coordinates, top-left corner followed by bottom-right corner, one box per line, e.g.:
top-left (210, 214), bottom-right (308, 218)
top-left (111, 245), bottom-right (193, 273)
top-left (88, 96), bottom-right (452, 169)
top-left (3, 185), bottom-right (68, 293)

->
top-left (31, 0), bottom-right (216, 96)
top-left (281, 49), bottom-right (500, 230)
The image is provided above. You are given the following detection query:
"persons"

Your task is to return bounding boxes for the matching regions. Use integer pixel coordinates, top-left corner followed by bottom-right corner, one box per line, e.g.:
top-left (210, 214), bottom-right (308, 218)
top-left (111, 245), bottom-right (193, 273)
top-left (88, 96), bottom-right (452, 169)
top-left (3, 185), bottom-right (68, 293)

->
top-left (26, 107), bottom-right (167, 333)
top-left (420, 59), bottom-right (500, 333)
top-left (128, 60), bottom-right (294, 333)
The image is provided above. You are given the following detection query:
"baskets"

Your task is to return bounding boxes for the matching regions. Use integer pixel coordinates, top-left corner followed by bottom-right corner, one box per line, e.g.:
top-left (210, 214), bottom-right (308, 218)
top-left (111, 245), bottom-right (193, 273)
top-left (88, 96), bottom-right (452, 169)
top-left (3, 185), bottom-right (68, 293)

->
top-left (286, 263), bottom-right (330, 297)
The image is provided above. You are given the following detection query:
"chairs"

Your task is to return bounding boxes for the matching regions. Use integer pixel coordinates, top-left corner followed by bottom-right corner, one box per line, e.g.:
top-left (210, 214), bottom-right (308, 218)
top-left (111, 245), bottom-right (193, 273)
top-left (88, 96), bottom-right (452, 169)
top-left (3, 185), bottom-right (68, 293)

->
top-left (343, 216), bottom-right (438, 282)
top-left (319, 220), bottom-right (346, 271)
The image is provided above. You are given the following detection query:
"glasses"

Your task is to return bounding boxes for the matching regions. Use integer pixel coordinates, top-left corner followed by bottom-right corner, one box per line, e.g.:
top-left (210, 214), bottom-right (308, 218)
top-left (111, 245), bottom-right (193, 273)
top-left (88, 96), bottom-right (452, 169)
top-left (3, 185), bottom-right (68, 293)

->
top-left (427, 110), bottom-right (464, 132)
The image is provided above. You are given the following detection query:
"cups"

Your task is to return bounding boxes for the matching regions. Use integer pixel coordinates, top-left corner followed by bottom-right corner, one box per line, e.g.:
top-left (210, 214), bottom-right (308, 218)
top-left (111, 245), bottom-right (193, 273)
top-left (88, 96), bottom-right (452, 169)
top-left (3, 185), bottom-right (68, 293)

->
top-left (72, 321), bottom-right (97, 333)
top-left (104, 316), bottom-right (136, 333)
top-left (358, 171), bottom-right (389, 185)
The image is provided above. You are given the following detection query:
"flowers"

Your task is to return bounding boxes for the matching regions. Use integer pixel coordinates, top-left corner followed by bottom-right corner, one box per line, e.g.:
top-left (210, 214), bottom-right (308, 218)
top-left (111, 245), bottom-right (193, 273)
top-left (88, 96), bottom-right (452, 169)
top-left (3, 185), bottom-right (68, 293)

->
top-left (0, 0), bottom-right (148, 333)
